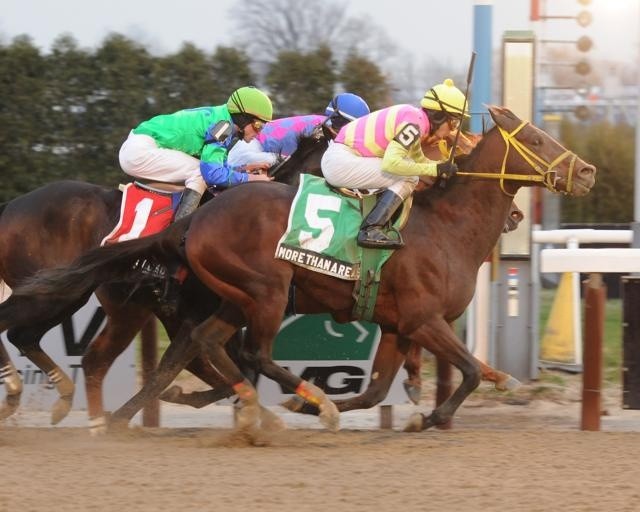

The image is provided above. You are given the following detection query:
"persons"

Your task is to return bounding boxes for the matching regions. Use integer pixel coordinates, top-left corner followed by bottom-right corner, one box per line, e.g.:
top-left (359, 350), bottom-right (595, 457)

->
top-left (118, 88), bottom-right (276, 228)
top-left (319, 79), bottom-right (471, 248)
top-left (230, 93), bottom-right (371, 181)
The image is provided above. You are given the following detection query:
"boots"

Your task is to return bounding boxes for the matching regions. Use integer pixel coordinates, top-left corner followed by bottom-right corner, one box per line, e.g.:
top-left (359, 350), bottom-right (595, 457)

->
top-left (357, 189), bottom-right (407, 249)
top-left (174, 188), bottom-right (202, 225)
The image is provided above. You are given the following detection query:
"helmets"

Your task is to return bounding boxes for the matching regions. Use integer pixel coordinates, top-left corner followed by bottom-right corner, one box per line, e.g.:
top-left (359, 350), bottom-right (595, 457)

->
top-left (227, 86), bottom-right (272, 122)
top-left (420, 78), bottom-right (472, 121)
top-left (325, 93), bottom-right (370, 122)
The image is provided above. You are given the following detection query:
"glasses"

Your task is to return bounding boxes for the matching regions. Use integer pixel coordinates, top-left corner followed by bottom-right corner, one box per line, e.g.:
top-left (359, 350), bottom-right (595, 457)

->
top-left (248, 117), bottom-right (265, 132)
top-left (445, 113), bottom-right (462, 130)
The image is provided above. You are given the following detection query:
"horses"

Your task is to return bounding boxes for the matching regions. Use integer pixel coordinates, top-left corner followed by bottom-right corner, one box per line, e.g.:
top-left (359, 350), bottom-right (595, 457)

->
top-left (0, 107), bottom-right (598, 444)
